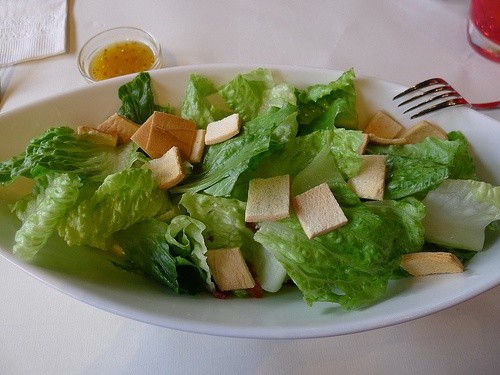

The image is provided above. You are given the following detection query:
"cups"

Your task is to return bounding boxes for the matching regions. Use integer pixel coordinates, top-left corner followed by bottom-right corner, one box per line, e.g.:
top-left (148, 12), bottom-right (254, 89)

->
top-left (466, 0), bottom-right (500, 63)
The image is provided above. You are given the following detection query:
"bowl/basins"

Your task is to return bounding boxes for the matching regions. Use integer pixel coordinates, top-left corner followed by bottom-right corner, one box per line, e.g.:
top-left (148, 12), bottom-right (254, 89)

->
top-left (76, 26), bottom-right (162, 84)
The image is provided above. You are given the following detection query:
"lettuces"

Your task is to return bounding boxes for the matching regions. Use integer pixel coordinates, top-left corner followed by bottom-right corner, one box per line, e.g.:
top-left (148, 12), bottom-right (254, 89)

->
top-left (0, 67), bottom-right (499, 311)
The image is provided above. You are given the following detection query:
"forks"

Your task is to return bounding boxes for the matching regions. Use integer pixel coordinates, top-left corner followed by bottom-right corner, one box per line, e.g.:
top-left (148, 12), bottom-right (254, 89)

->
top-left (393, 78), bottom-right (499, 120)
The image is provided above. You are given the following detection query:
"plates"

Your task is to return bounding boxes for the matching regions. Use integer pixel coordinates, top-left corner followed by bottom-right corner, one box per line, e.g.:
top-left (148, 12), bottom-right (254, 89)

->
top-left (1, 64), bottom-right (499, 340)
